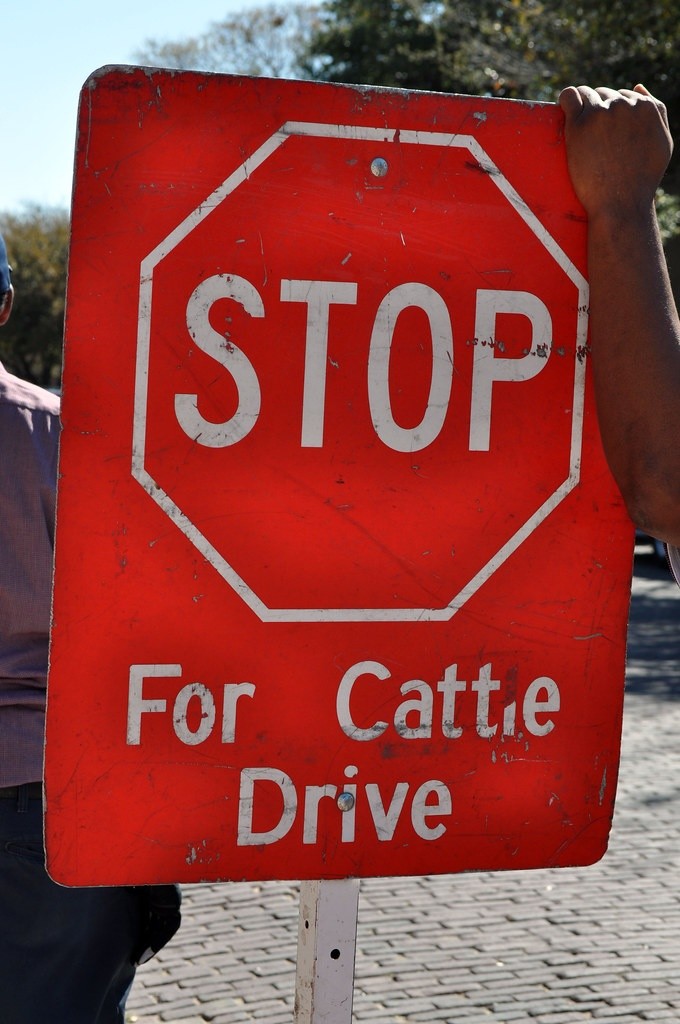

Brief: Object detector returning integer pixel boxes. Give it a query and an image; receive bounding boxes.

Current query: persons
[0,236,180,1024]
[562,82,680,585]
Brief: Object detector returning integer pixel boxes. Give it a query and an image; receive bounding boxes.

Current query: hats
[0,235,13,294]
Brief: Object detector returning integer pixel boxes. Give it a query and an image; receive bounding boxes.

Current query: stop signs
[132,124,593,624]
